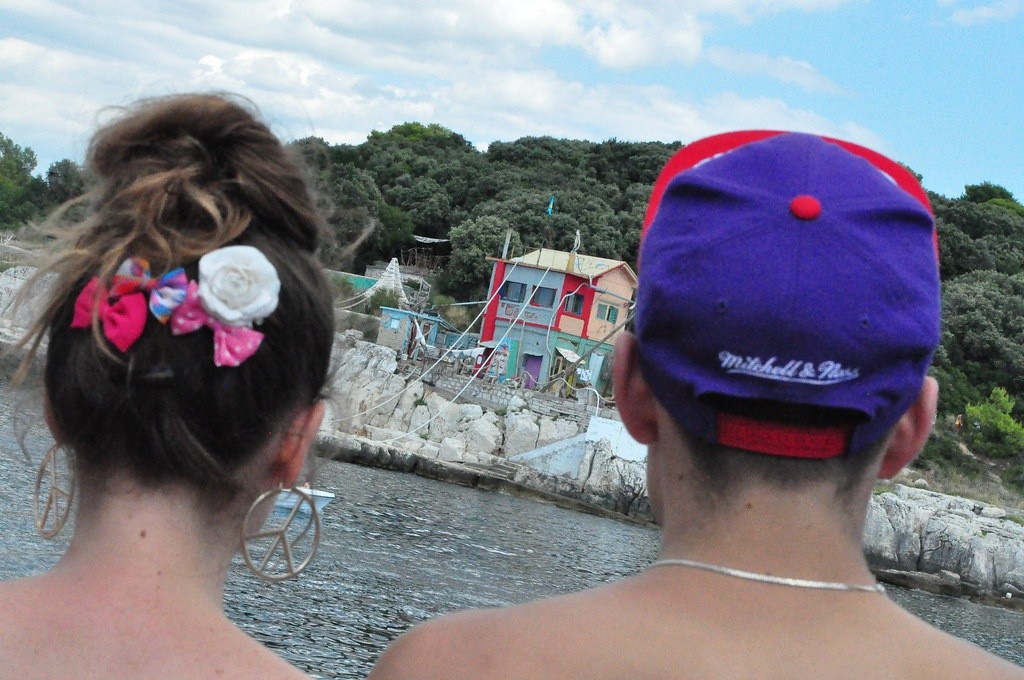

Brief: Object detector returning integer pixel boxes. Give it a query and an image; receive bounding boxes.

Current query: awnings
[556,347,585,364]
[479,340,498,348]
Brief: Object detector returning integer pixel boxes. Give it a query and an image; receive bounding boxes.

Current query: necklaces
[653,560,884,592]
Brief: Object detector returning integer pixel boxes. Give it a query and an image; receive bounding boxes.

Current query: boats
[274,486,336,516]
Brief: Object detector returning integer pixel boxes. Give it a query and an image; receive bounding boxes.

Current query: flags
[548,197,554,215]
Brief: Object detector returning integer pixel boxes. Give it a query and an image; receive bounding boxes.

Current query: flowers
[196,244,283,330]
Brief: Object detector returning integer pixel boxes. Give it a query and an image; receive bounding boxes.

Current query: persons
[370,129,1024,680]
[0,93,334,680]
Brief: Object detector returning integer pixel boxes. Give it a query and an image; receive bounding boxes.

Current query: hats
[636,130,941,457]
